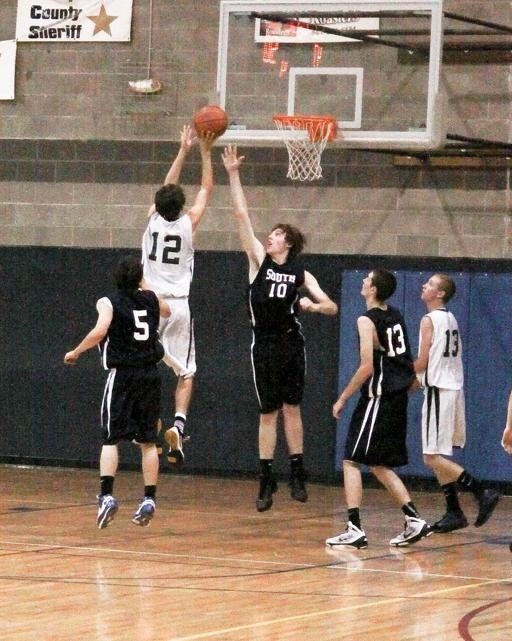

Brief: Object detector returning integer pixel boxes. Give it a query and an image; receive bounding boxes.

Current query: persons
[63,255,172,530]
[131,124,219,465]
[220,141,340,512]
[500,388,512,457]
[324,266,435,550]
[412,271,500,534]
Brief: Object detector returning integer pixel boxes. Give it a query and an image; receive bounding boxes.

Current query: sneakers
[430,511,470,534]
[471,485,500,529]
[325,522,369,550]
[254,477,278,513]
[164,425,187,470]
[154,416,163,459]
[96,493,117,530]
[129,496,156,528]
[289,473,310,502]
[388,513,433,548]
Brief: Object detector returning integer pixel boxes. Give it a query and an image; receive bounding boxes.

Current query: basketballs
[195,106,227,137]
